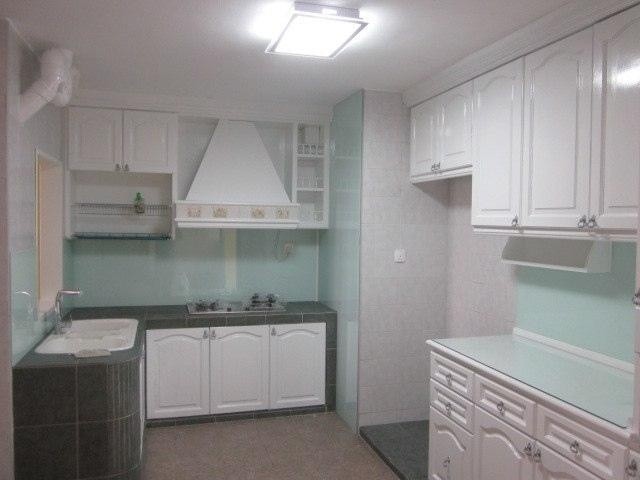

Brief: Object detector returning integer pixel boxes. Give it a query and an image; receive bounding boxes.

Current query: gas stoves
[185,290,286,315]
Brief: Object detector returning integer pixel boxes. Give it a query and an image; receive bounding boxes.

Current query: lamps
[263,3,369,60]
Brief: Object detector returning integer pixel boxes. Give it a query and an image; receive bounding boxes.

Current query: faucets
[14,288,34,326]
[54,290,81,335]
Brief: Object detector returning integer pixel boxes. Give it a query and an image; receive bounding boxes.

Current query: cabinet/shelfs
[271,325,326,410]
[475,372,627,480]
[290,120,330,228]
[147,324,270,419]
[409,80,471,183]
[472,57,519,230]
[65,171,175,244]
[521,3,639,233]
[426,351,474,478]
[67,105,179,174]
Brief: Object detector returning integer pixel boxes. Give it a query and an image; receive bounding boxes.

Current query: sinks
[35,334,134,354]
[74,318,139,333]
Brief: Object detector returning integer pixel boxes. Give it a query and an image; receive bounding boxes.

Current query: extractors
[174,117,300,230]
